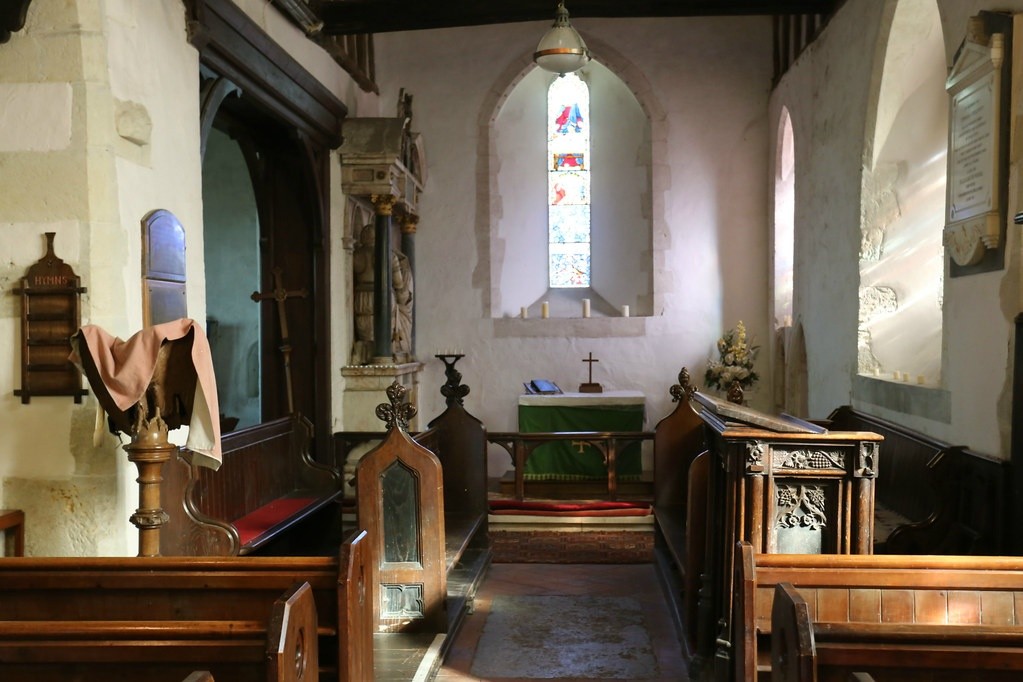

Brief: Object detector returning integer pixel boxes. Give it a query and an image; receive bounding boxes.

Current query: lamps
[534,0,594,77]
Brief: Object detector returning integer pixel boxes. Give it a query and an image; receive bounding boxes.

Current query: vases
[728,380,744,404]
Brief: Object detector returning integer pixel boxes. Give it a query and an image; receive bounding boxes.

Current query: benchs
[159,413,342,558]
[652,368,717,682]
[355,371,488,634]
[0,529,375,682]
[735,541,1023,681]
[816,407,964,555]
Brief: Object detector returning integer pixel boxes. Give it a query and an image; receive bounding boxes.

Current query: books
[531,380,555,395]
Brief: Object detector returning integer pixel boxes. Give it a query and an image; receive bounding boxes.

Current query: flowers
[703,320,762,391]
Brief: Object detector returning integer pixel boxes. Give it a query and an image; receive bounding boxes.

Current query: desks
[518,390,647,481]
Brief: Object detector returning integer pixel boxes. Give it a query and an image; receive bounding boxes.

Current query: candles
[542,301,549,318]
[436,349,464,355]
[521,307,527,318]
[582,299,591,318]
[621,305,629,317]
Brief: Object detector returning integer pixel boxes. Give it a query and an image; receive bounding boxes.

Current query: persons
[351,224,414,366]
